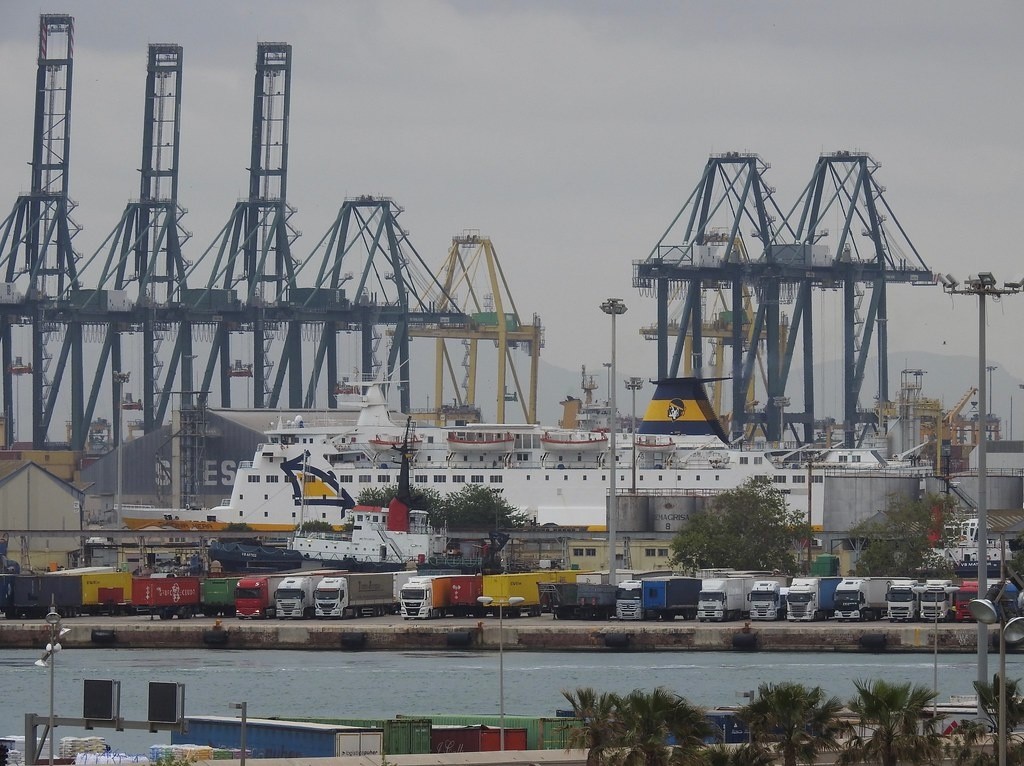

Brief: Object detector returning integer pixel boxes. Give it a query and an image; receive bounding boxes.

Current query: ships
[111,376,935,557]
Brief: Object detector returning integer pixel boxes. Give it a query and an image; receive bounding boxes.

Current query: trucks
[616,579,661,621]
[884,579,1020,623]
[785,576,844,622]
[536,582,616,622]
[745,580,790,622]
[832,576,917,622]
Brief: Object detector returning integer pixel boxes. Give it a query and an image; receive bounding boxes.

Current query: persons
[478,542,489,564]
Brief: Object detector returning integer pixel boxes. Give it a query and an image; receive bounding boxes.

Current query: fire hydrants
[216,619,223,628]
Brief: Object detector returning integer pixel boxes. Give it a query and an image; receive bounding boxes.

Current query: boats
[632,436,678,452]
[538,431,610,454]
[446,430,516,453]
[368,436,423,451]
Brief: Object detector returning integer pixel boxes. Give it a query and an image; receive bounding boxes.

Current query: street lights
[599,297,628,586]
[478,596,524,751]
[772,395,790,447]
[987,364,998,434]
[940,271,1024,724]
[965,580,1021,766]
[800,448,822,565]
[624,376,645,487]
[601,361,614,403]
[35,606,71,766]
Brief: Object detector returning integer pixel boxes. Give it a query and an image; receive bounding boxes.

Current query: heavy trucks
[232,569,348,621]
[313,570,420,620]
[273,575,326,619]
[641,575,707,622]
[697,577,788,626]
[400,568,598,620]
